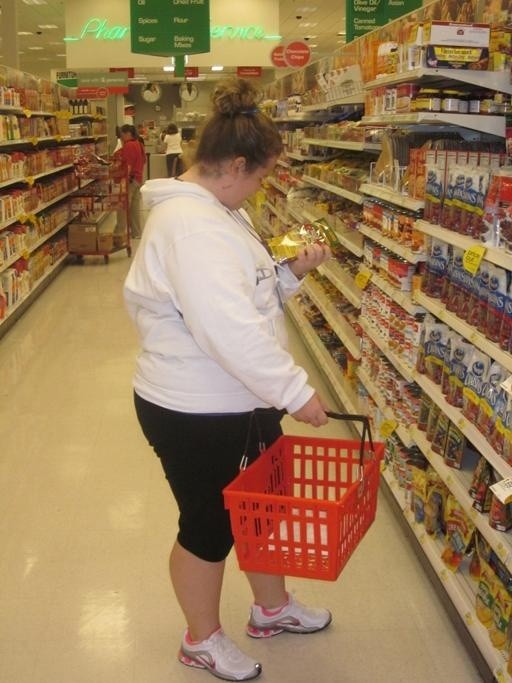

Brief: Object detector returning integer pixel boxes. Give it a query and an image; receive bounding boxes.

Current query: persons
[113,123,184,239]
[121,78,332,681]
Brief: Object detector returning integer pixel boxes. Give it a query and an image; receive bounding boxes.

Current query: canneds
[410,86,511,116]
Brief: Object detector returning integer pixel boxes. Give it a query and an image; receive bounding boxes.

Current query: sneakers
[245,590,332,639]
[176,622,264,682]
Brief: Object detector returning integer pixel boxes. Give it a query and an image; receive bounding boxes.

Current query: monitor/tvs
[182,127,196,142]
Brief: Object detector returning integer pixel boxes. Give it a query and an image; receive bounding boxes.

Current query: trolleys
[65,156,132,263]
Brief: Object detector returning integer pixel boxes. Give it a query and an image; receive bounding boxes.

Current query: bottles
[360,196,426,255]
[68,96,95,137]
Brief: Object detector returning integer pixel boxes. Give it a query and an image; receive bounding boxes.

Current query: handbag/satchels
[218,402,389,583]
